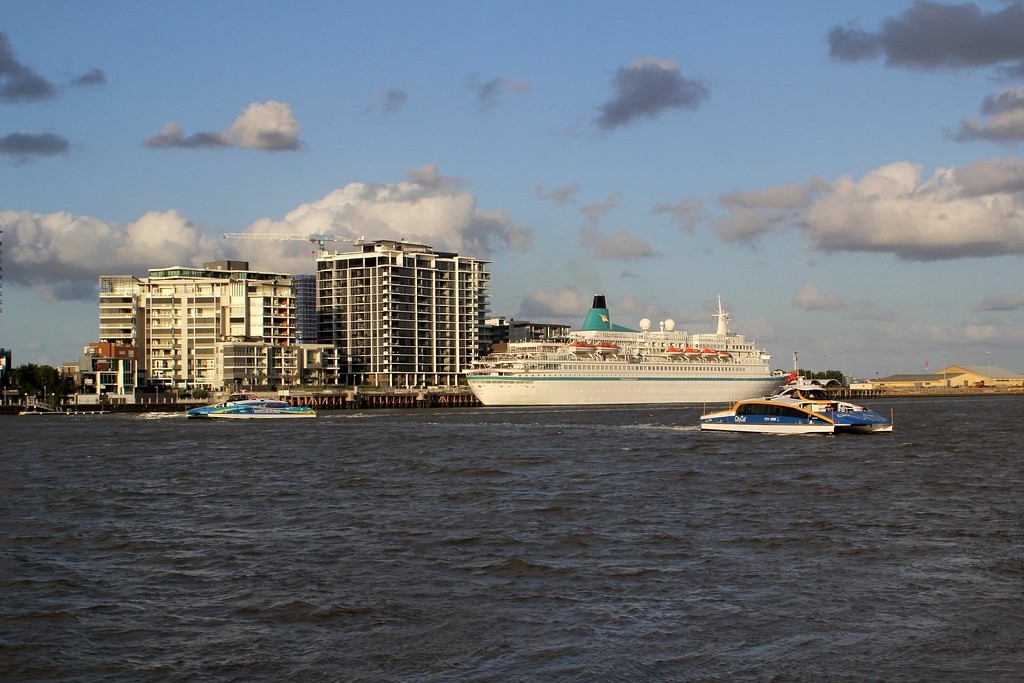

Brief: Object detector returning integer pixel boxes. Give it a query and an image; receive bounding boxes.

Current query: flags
[784,372,796,382]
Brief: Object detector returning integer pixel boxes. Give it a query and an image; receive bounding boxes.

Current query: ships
[459,294,797,408]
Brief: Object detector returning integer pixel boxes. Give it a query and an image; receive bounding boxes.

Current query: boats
[663,347,683,355]
[702,348,718,357]
[185,391,317,419]
[699,370,895,437]
[594,342,621,354]
[568,341,596,355]
[719,351,730,358]
[684,349,701,359]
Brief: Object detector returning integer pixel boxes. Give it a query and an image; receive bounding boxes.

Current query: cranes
[220,229,354,256]
